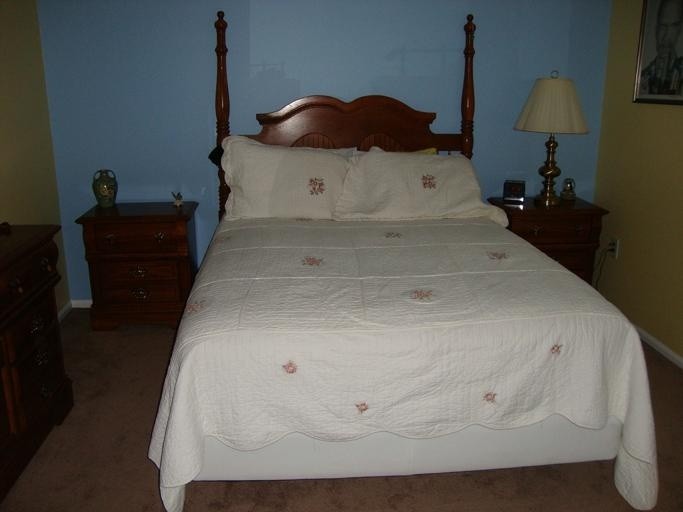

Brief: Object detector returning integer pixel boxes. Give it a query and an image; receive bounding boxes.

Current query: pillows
[220,133,511,226]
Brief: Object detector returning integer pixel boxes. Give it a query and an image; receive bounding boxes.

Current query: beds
[165,9,648,512]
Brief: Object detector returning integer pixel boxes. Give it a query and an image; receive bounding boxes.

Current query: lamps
[510,69,591,208]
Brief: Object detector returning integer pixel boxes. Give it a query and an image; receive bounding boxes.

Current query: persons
[638,0,682,98]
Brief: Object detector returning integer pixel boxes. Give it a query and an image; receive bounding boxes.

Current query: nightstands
[484,193,610,276]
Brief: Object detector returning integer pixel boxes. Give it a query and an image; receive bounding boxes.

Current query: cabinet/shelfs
[1,219,76,508]
[75,198,198,328]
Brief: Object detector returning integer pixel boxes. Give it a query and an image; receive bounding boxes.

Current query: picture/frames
[629,0,682,107]
